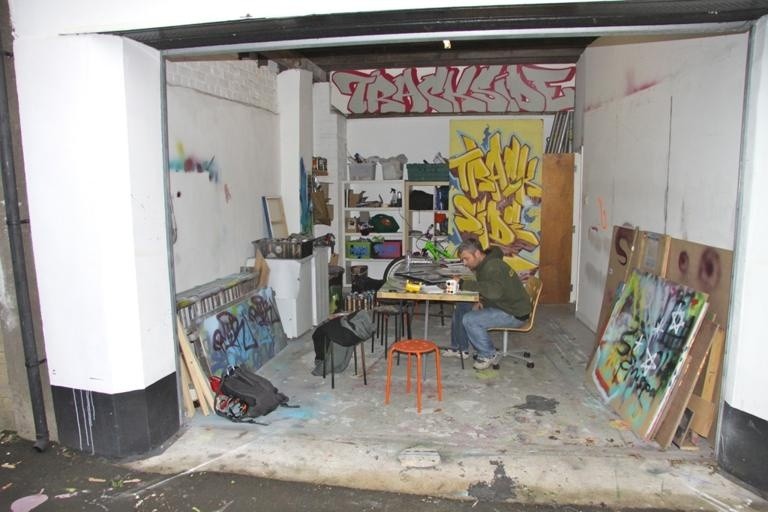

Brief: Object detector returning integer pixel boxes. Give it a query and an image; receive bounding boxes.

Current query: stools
[383,336,442,412]
[370,302,415,354]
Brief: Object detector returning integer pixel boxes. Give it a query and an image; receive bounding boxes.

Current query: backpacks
[213,365,300,427]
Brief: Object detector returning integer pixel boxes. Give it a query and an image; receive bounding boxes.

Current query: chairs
[482,273,545,372]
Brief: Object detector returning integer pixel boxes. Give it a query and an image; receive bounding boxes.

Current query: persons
[442,239,532,370]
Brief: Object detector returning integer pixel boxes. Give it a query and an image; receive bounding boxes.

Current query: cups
[445,279,460,295]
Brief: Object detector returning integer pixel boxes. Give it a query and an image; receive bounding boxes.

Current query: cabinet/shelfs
[342,179,450,290]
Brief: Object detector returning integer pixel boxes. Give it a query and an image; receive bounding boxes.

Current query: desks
[375,268,479,368]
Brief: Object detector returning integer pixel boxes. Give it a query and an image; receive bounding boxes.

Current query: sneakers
[439,347,471,360]
[471,349,500,370]
